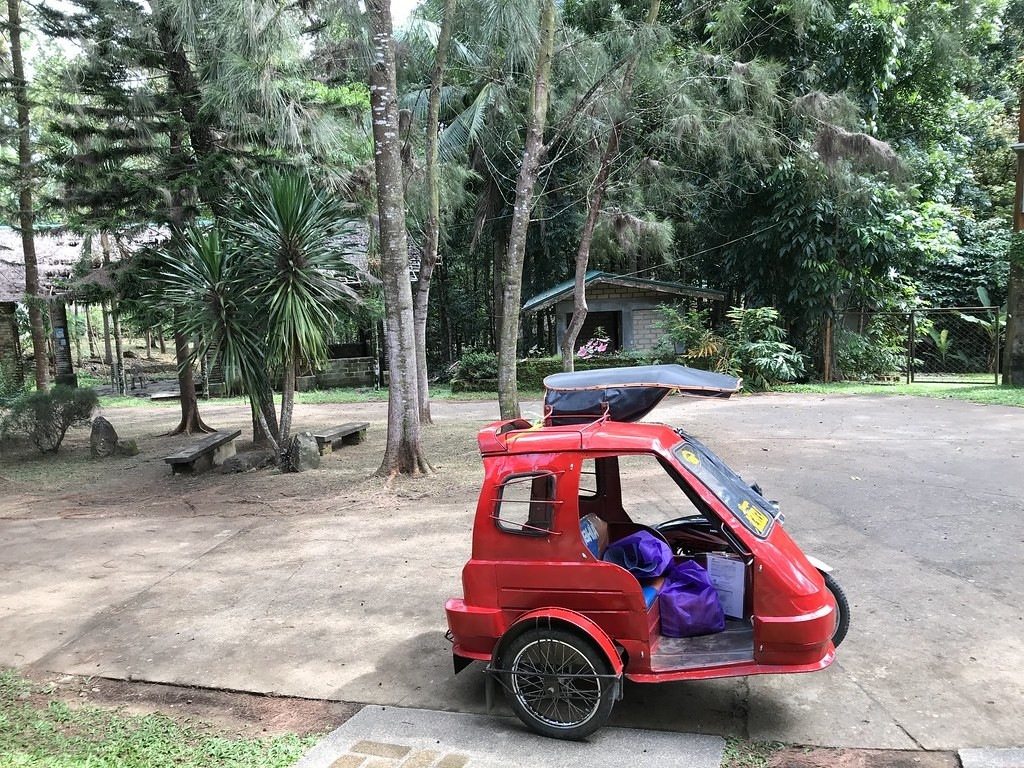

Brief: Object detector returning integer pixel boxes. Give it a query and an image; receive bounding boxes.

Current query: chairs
[579,513,665,612]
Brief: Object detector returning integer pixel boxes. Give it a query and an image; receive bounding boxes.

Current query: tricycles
[445,363,852,743]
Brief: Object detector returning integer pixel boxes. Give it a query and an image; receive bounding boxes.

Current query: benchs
[165,430,241,475]
[313,422,370,456]
